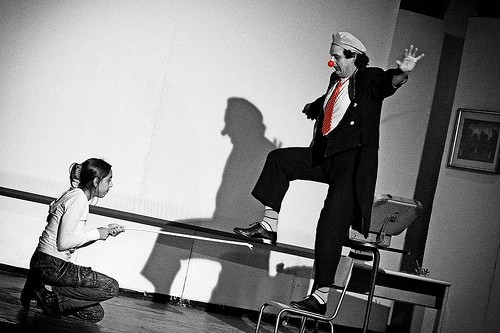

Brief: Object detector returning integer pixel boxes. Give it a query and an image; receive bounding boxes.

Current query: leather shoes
[233,223,277,247]
[291,295,328,316]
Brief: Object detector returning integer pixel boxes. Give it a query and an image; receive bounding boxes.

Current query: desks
[348,263,451,333]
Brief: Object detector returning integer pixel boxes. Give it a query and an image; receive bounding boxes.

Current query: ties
[321,77,348,135]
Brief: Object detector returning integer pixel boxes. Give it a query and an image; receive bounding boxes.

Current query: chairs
[255,256,354,333]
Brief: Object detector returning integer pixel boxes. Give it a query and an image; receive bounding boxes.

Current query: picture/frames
[446,108,500,174]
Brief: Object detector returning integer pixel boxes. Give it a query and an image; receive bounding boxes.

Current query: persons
[20,158,124,323]
[447,108,500,175]
[232,32,424,315]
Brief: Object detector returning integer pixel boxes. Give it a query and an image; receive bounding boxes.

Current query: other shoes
[20,273,40,310]
[32,283,53,314]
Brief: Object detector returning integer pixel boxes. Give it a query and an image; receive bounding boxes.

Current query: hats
[332,32,368,54]
